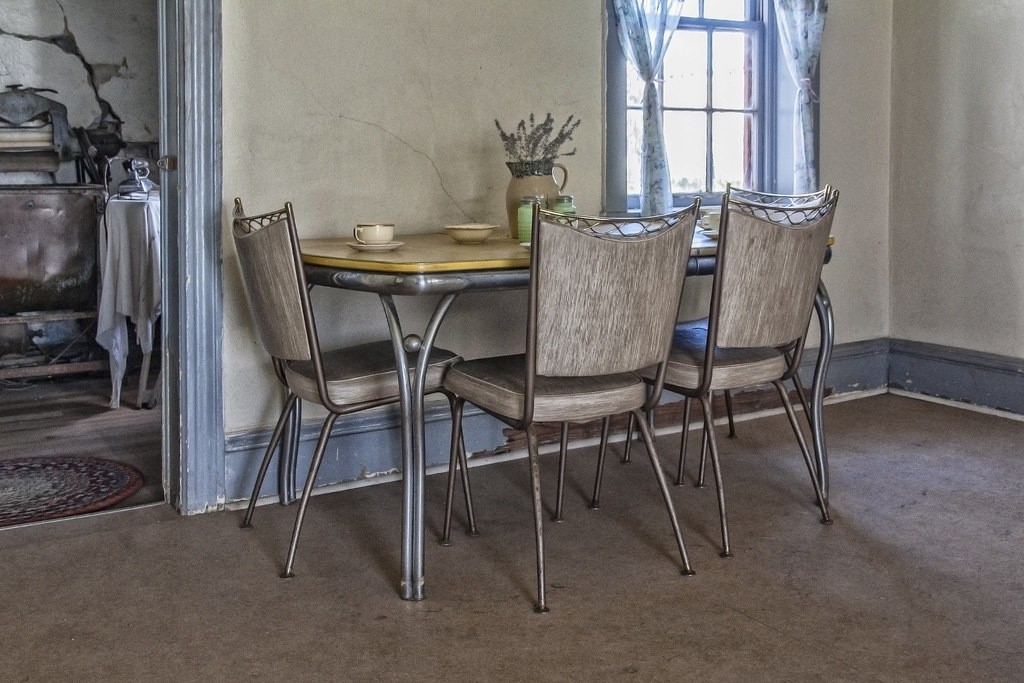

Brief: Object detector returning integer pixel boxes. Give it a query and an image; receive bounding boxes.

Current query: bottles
[551,196,577,214]
[518,196,542,242]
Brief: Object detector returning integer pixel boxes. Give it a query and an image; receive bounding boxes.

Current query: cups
[354,223,395,244]
[701,212,722,230]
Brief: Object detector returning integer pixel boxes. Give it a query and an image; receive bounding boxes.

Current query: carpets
[0,455,145,526]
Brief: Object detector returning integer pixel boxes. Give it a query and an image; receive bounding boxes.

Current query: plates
[700,230,719,239]
[519,242,531,249]
[345,240,405,253]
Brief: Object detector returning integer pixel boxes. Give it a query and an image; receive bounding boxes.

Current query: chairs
[441,195,702,614]
[619,183,834,489]
[591,178,843,559]
[230,195,481,579]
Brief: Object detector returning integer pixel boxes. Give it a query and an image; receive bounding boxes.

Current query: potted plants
[493,112,584,239]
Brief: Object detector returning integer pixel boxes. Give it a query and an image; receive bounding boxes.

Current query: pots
[1,84,59,148]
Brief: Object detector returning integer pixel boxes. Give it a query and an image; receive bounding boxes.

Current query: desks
[94,194,161,408]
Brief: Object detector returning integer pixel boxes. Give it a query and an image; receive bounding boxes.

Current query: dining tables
[279,223,835,601]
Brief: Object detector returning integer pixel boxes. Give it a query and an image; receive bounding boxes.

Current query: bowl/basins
[444,224,498,245]
